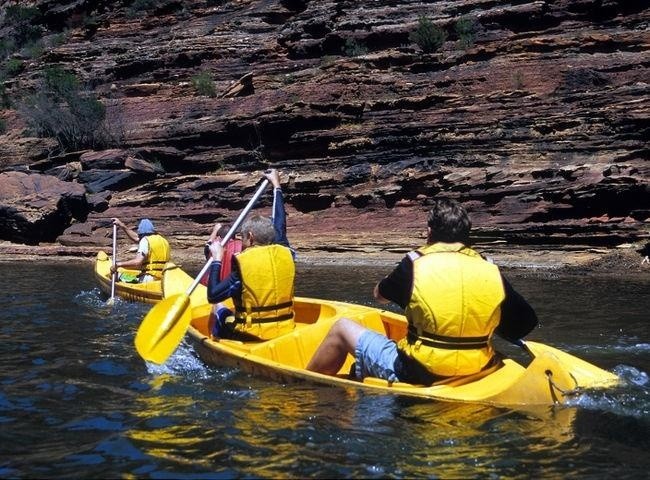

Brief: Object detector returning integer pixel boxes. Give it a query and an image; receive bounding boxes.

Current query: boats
[161,263,625,411]
[94,250,162,305]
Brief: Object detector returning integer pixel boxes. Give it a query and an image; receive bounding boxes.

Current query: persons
[199,224,244,288]
[207,168,298,340]
[109,216,170,284]
[305,201,538,385]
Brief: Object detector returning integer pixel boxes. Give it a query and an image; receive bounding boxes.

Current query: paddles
[134,170,270,366]
[490,333,535,368]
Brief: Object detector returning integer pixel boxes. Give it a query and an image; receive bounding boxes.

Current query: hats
[137,218,156,234]
